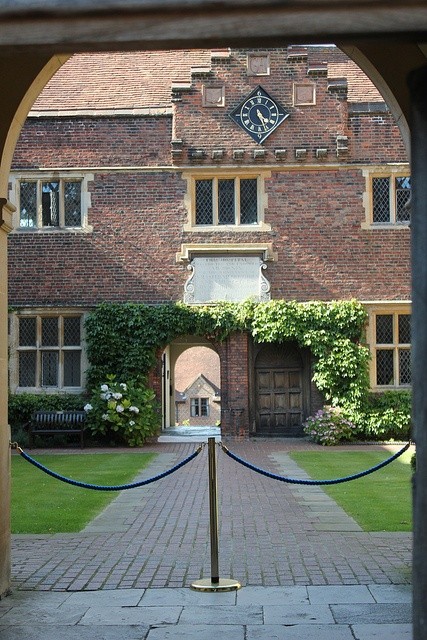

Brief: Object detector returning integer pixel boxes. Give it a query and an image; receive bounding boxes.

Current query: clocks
[228,84,291,146]
